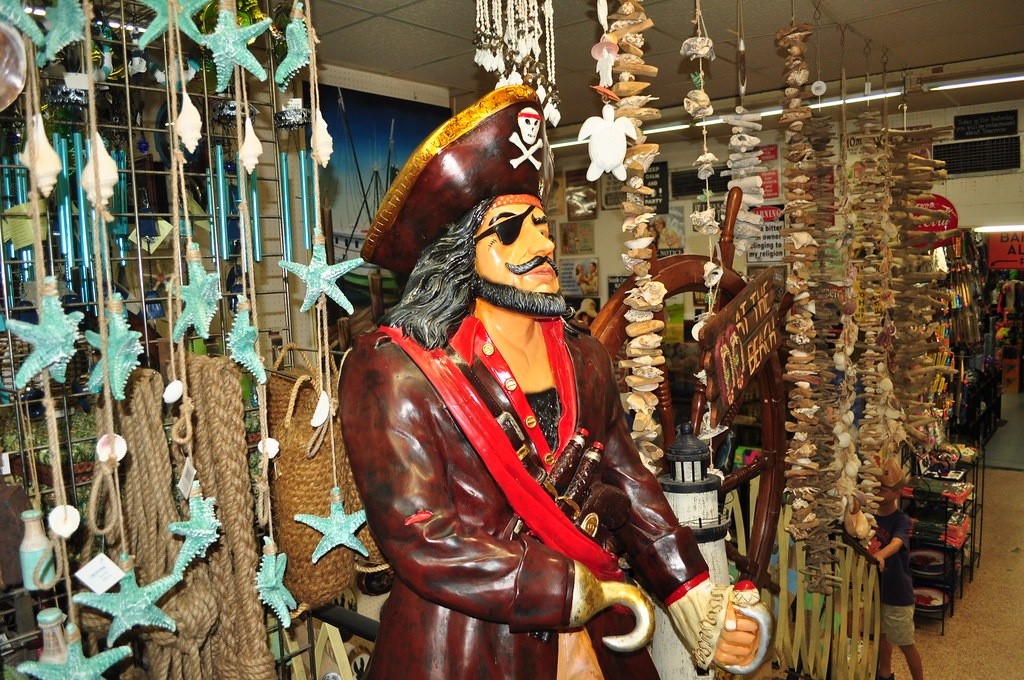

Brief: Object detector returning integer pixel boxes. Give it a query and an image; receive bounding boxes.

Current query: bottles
[973,306,981,342]
[942,325,949,349]
[941,319,947,338]
[948,402,954,420]
[950,319,958,347]
[963,309,973,341]
[966,265,984,305]
[961,266,971,307]
[973,266,981,290]
[942,353,954,380]
[956,268,963,308]
[950,268,956,308]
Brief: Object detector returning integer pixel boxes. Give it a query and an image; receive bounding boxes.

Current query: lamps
[922,69,1024,93]
[548,86,906,149]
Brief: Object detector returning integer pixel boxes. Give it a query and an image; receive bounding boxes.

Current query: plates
[909,549,945,575]
[913,588,949,612]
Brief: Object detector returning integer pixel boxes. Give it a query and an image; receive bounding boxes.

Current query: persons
[870,485,927,680]
[337,81,776,680]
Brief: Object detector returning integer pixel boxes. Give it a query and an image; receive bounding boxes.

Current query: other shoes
[879,672,894,680]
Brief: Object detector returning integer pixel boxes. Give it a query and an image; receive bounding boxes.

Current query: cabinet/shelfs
[899,445,986,634]
[947,363,1003,448]
